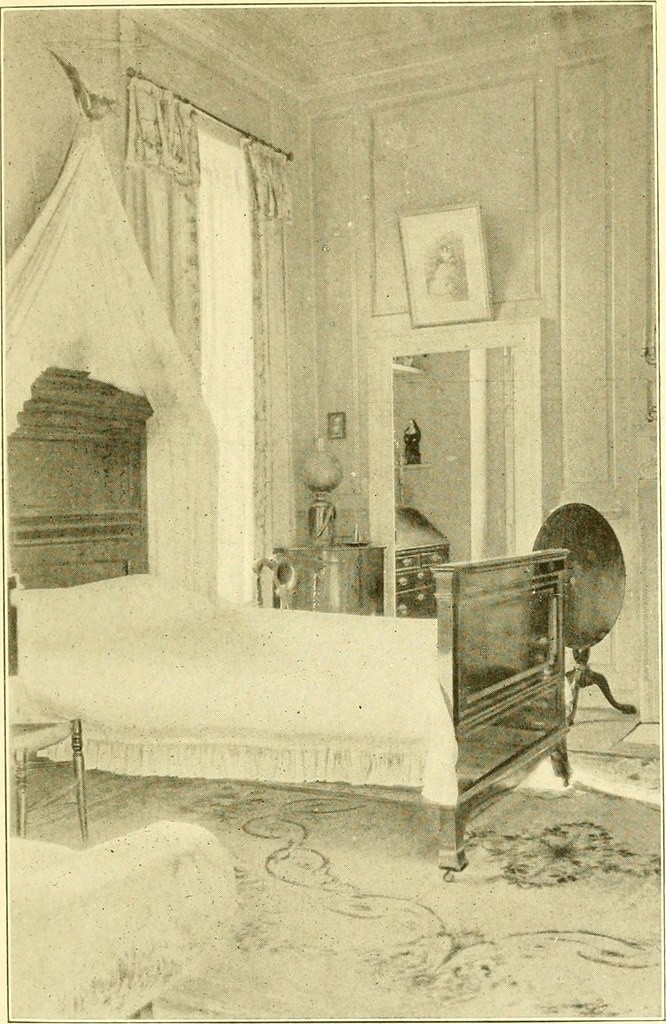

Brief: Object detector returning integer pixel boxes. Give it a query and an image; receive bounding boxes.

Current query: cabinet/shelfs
[269,544,385,619]
[394,507,449,619]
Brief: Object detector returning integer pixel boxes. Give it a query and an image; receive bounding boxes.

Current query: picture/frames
[327,411,347,440]
[396,204,495,328]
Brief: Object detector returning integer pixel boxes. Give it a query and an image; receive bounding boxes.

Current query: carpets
[8,754,664,1020]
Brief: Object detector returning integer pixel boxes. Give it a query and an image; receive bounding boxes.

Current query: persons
[403,419,421,458]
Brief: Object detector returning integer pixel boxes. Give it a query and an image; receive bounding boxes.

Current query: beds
[4,364,575,867]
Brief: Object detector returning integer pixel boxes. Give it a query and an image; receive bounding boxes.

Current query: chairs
[4,572,90,844]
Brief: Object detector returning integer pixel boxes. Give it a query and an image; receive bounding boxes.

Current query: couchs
[8,817,240,1022]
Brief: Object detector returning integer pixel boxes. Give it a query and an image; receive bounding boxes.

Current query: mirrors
[363,316,542,621]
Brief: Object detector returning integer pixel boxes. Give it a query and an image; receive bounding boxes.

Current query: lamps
[298,451,342,548]
